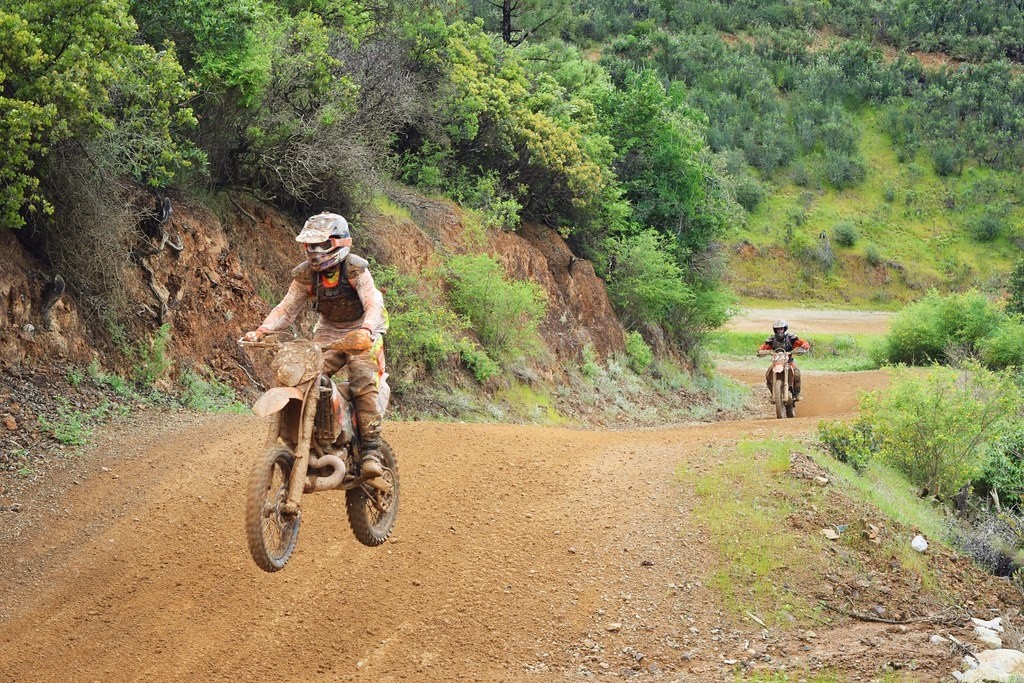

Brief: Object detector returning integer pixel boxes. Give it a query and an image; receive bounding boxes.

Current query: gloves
[243,330,263,351]
[344,329,371,355]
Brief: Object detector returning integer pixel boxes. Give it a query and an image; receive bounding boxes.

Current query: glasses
[307,238,353,253]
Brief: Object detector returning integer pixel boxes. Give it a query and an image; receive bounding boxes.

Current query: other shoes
[794,393,803,401]
[361,449,382,477]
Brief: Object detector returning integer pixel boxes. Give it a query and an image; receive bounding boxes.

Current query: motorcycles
[756,349,805,419]
[237,330,402,570]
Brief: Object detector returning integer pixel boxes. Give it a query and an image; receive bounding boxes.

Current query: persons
[757,320,810,401]
[243,211,389,479]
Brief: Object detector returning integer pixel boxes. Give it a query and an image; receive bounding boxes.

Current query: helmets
[773,319,788,339]
[296,213,352,271]
